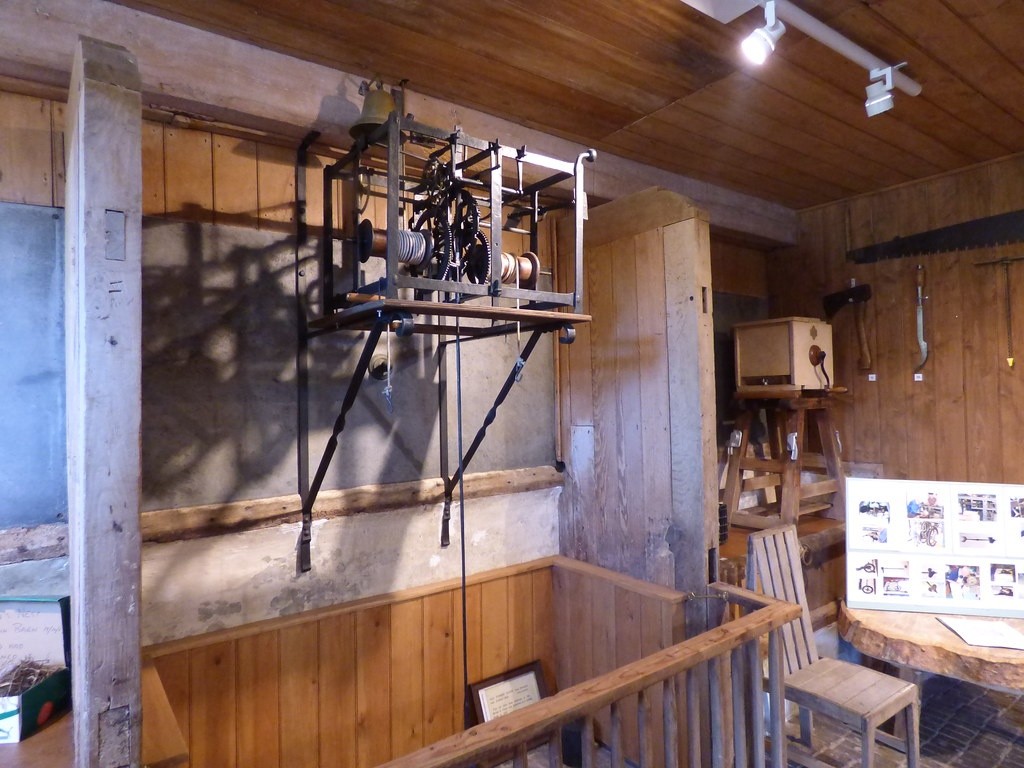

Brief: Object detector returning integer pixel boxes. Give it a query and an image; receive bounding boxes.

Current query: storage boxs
[0,592,71,746]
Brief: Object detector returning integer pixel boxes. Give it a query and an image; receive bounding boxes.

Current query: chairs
[744,526,921,768]
